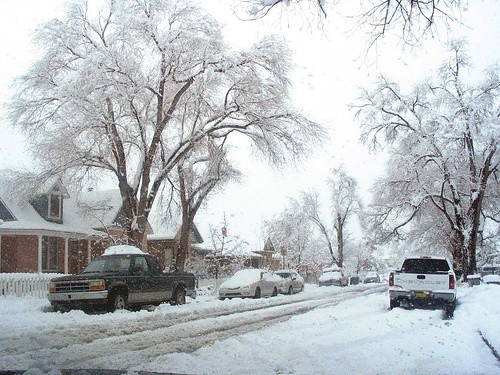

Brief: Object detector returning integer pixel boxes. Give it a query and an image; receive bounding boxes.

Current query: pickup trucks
[47,253,199,313]
[388,255,457,313]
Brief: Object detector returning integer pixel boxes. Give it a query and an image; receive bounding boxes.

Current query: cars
[363,273,381,283]
[319,267,350,288]
[274,269,305,296]
[218,268,281,298]
